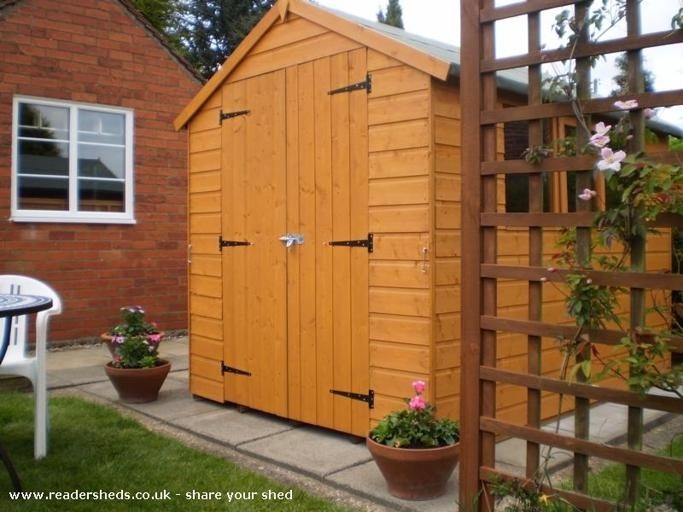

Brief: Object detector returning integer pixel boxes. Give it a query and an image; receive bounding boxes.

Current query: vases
[102,331,161,357]
[366,432,459,500]
[105,360,171,404]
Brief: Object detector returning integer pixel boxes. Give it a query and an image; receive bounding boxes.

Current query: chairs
[0,275,62,461]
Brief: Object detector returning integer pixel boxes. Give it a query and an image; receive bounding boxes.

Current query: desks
[0,294,53,497]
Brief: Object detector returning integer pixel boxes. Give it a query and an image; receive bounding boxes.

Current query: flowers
[370,381,459,448]
[107,306,159,337]
[112,334,161,369]
